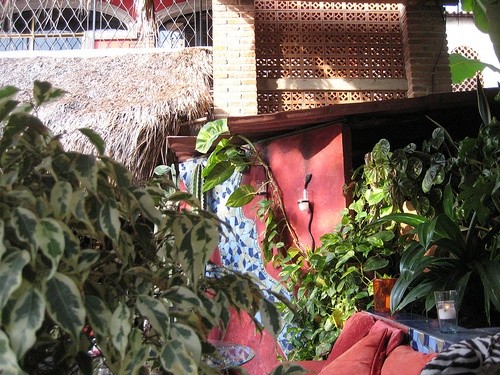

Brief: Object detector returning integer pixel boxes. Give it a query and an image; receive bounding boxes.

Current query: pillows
[311,312,438,374]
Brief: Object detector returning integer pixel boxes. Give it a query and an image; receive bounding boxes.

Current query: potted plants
[372,271,399,317]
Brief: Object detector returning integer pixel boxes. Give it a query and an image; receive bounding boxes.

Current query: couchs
[208,299,326,375]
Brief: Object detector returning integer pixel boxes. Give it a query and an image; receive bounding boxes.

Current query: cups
[372,278,396,314]
[434,291,459,334]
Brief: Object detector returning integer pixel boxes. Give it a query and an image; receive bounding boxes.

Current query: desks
[360,309,497,354]
[201,339,255,375]
